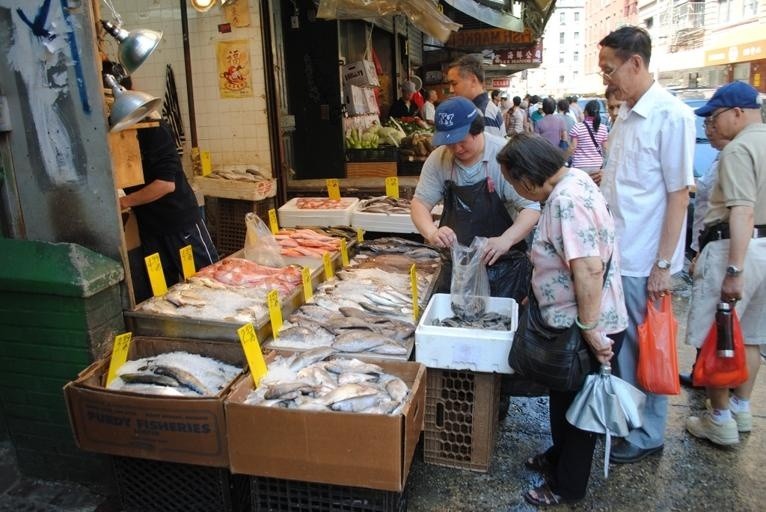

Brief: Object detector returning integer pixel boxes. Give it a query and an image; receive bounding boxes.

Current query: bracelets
[573,315,603,331]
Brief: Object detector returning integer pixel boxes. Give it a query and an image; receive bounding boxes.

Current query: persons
[692,79,766,448]
[490,89,500,106]
[500,94,506,111]
[448,55,508,138]
[496,133,630,506]
[599,25,698,465]
[572,97,583,118]
[681,117,729,384]
[534,97,567,147]
[531,104,545,129]
[561,100,607,173]
[506,96,527,136]
[528,95,542,115]
[423,89,438,125]
[388,82,428,128]
[409,97,541,298]
[604,89,624,124]
[519,93,529,108]
[566,96,583,120]
[96,60,221,289]
[554,99,576,134]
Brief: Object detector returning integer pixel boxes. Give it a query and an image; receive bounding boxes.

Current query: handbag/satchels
[508,202,615,393]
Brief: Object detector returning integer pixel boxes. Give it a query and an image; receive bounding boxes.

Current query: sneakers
[686,415,740,446]
[706,397,753,433]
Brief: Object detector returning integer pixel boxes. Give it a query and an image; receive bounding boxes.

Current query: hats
[694,80,761,117]
[402,81,416,92]
[431,95,479,146]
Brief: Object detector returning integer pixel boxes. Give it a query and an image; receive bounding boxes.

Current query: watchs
[656,259,672,269]
[725,266,745,275]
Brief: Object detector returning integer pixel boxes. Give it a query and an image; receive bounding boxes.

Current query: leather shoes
[678,373,703,390]
[610,438,664,464]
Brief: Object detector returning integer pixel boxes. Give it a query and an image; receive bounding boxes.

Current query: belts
[707,225,765,240]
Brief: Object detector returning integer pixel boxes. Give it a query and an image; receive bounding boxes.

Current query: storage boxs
[214,197,268,259]
[223,349,427,493]
[345,142,396,162]
[249,475,407,511]
[277,196,360,227]
[107,454,251,512]
[61,335,264,470]
[344,84,365,116]
[341,60,381,88]
[423,366,503,472]
[362,87,381,115]
[413,292,521,376]
[196,174,278,202]
[351,199,443,233]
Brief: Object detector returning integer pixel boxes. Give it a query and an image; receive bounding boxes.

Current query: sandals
[525,446,554,469]
[526,479,586,505]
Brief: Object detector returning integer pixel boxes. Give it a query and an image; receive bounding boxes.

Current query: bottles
[715,301,735,357]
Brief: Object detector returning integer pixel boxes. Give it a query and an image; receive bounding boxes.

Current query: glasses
[598,57,629,81]
[707,106,744,125]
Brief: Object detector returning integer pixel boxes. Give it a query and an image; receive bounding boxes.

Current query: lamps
[189,0,217,12]
[98,17,163,77]
[103,72,161,134]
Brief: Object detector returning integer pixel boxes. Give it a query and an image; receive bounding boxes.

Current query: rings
[660,293,665,297]
[729,298,737,302]
